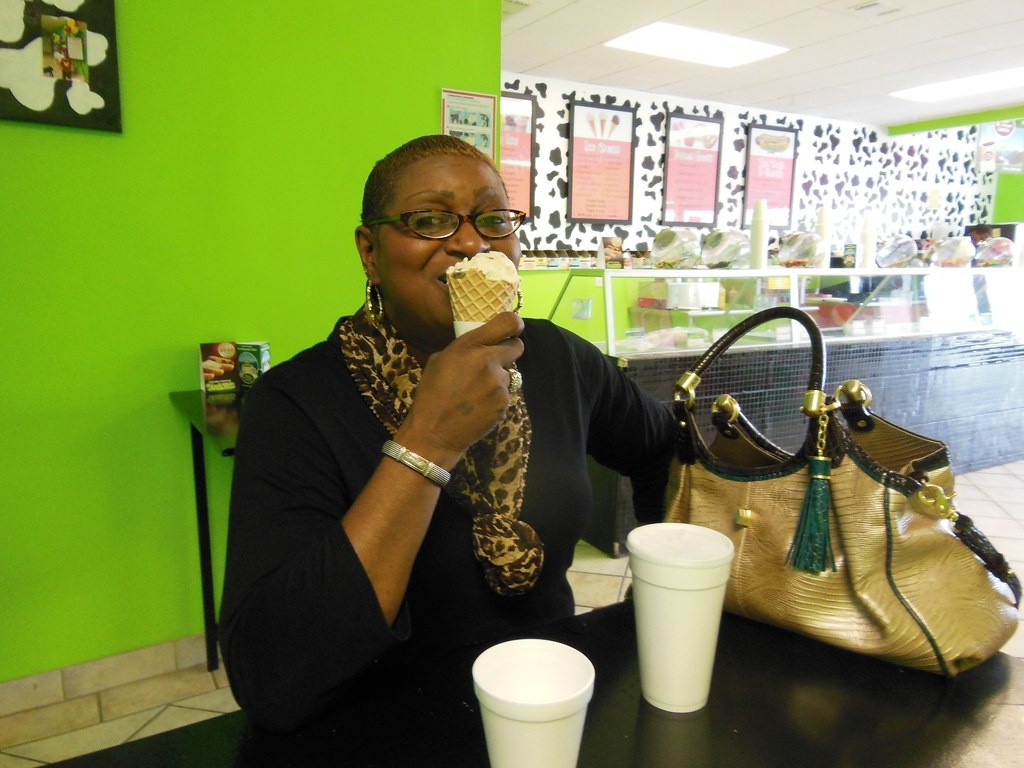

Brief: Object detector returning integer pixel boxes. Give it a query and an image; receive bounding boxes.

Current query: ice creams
[582,111,620,138]
[447,251,518,323]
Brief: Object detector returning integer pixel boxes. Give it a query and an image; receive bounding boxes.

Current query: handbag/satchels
[663,307,1022,675]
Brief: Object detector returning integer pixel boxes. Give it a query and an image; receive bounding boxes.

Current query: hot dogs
[756,133,790,152]
[201,356,234,379]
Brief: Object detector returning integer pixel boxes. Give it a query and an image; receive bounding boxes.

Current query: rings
[508,369,523,392]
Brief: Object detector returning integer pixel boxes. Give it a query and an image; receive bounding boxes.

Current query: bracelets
[383,440,451,488]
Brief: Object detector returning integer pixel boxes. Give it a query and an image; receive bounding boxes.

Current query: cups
[750,199,769,269]
[812,205,830,269]
[472,638,596,767]
[626,523,735,714]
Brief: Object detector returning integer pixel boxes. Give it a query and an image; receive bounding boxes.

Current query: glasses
[363,208,526,239]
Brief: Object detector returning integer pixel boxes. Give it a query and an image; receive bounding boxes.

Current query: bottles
[778,231,825,269]
[701,228,748,270]
[650,229,701,269]
[875,234,1016,269]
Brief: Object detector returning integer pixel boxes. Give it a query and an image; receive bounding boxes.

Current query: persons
[967,224,995,289]
[217,134,681,731]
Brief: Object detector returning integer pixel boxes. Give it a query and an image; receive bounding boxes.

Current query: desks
[32,593,1024,768]
[168,388,245,675]
